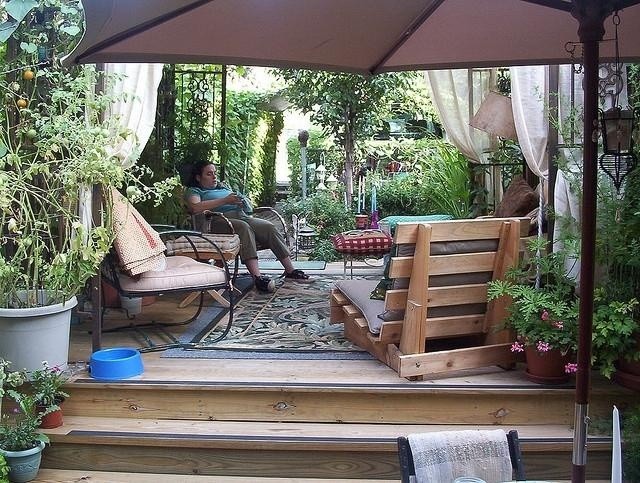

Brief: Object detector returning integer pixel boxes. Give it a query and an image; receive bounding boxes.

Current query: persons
[184,160,316,293]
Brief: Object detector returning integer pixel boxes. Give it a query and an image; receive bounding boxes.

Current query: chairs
[328,216,532,383]
[472,174,544,237]
[397,428,525,483]
[88,183,233,353]
[181,206,291,288]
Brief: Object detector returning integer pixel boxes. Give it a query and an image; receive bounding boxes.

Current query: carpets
[227,260,326,271]
[159,273,387,360]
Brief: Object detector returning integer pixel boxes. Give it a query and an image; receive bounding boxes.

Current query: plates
[300,230,314,233]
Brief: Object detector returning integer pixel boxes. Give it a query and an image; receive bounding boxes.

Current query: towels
[407,428,513,483]
[107,185,168,277]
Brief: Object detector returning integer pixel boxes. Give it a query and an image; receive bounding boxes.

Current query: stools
[332,229,392,279]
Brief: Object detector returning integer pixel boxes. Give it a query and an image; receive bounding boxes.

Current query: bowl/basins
[89,348,144,380]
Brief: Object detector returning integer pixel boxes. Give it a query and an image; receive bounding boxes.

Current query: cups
[356,215,368,230]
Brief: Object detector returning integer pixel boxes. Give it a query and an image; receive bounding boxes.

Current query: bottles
[379,219,390,235]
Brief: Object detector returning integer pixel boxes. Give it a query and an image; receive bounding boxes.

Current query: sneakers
[282,269,316,284]
[252,275,275,292]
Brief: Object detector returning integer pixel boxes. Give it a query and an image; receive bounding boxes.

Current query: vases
[35,396,65,429]
[519,335,565,378]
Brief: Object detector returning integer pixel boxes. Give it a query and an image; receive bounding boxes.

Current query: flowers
[486,239,578,381]
[22,359,68,407]
[0,355,31,417]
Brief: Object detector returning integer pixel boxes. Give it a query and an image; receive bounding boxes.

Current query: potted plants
[0,65,140,382]
[590,285,639,380]
[0,404,62,483]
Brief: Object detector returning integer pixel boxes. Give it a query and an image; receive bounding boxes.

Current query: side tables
[377,213,455,276]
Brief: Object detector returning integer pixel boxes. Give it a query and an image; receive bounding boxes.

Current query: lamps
[467,89,518,141]
[295,212,315,261]
[591,14,639,195]
